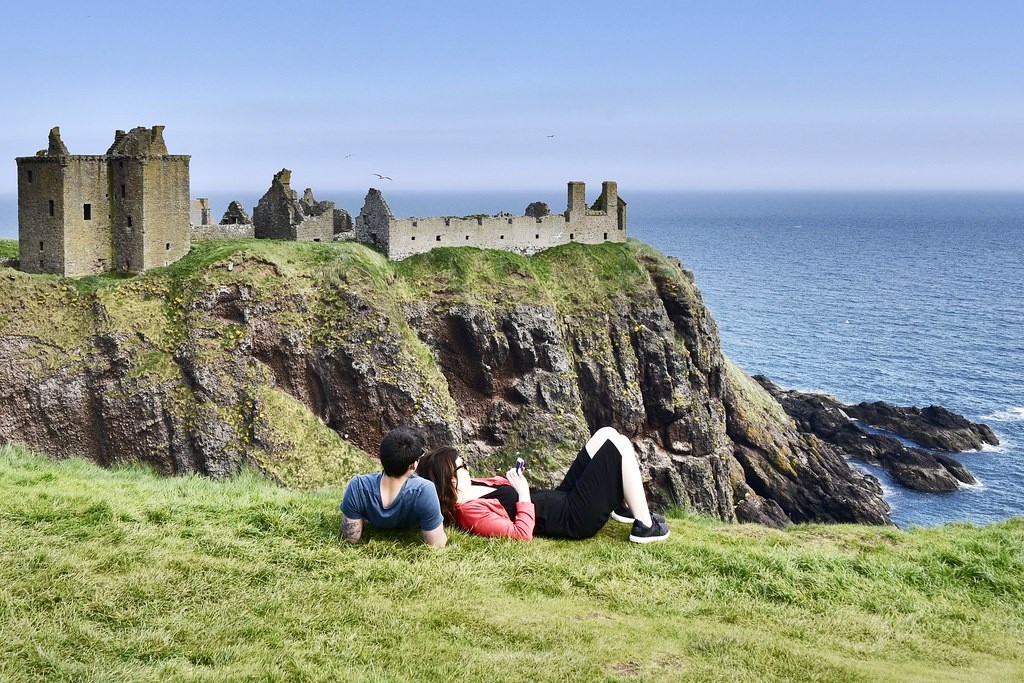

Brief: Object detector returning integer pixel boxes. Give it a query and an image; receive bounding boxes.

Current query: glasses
[417,448,426,461]
[453,459,467,473]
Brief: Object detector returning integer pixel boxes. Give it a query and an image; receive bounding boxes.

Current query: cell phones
[515,458,524,475]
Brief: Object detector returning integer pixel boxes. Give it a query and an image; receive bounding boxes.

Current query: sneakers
[610,502,667,525]
[629,519,671,544]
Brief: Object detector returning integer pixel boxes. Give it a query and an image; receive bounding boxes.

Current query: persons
[340,427,447,548]
[417,427,671,543]
